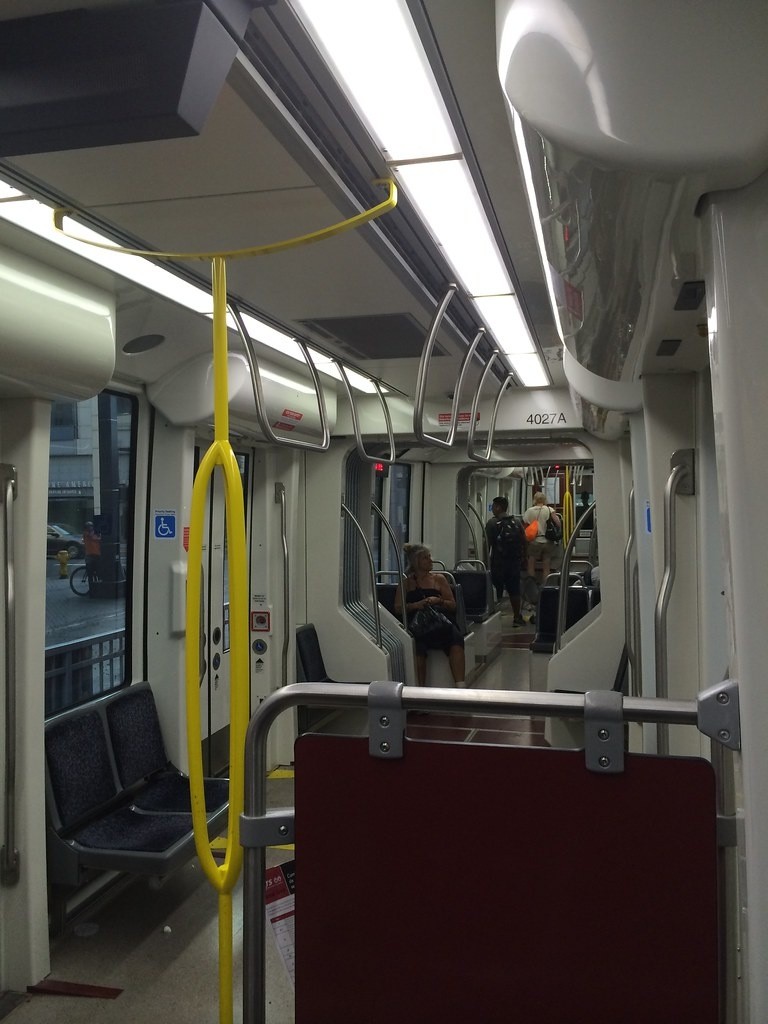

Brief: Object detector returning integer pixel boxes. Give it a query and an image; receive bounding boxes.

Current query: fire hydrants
[58,550,69,579]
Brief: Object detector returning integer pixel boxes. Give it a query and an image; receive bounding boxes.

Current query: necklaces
[418,573,429,581]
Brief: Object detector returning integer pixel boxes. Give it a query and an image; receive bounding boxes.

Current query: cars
[47,522,85,560]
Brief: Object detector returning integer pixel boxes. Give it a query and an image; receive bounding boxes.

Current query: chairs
[46,554,601,923]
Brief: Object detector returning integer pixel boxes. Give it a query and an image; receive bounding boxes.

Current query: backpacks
[495,514,524,563]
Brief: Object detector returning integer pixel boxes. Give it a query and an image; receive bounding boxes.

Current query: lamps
[2,183,388,395]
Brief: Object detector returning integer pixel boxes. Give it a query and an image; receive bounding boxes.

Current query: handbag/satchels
[409,599,454,643]
[524,520,538,542]
[545,506,564,541]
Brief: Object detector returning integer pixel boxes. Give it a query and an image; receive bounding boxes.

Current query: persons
[394,542,467,689]
[483,496,531,626]
[576,491,594,529]
[82,521,101,594]
[522,490,562,585]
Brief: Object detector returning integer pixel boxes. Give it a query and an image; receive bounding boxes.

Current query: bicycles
[70,562,98,597]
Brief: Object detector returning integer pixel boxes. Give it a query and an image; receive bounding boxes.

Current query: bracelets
[439,595,444,607]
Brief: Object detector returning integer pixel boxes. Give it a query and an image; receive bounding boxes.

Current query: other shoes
[512,614,527,627]
